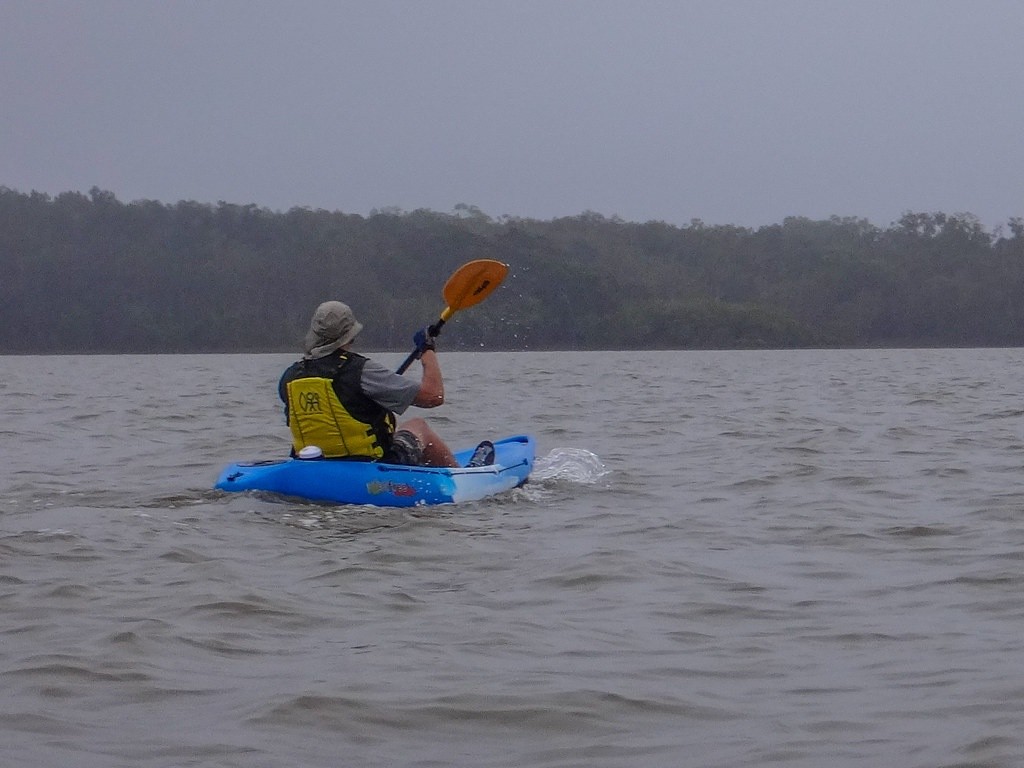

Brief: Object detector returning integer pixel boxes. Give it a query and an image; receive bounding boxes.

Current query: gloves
[413,325,436,360]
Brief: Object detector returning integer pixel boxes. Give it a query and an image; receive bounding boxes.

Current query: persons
[278,300,496,471]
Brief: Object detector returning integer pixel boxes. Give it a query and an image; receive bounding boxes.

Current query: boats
[212,434,537,509]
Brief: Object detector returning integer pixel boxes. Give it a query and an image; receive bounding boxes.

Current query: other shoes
[464,440,495,468]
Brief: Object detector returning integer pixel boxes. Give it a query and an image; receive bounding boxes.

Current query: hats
[303,301,363,359]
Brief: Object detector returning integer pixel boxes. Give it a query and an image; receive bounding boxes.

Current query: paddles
[394,258,509,375]
